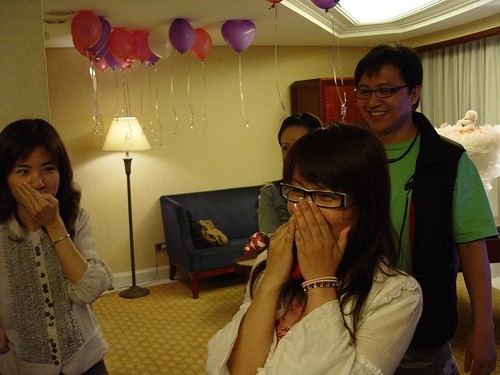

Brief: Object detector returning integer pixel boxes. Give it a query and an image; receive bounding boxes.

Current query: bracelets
[53,233,71,247]
[302,276,339,291]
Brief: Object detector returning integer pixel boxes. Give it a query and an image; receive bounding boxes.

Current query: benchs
[160,185,263,299]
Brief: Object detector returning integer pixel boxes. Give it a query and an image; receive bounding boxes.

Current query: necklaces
[388,130,418,164]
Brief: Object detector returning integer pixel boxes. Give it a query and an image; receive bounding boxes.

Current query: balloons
[73,37,132,71]
[87,16,110,53]
[266,0,283,3]
[190,28,212,59]
[311,0,340,9]
[71,10,101,47]
[169,18,195,54]
[221,20,257,53]
[108,27,133,66]
[147,24,175,58]
[144,52,159,64]
[132,30,148,62]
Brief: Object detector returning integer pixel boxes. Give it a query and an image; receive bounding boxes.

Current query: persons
[0,117,114,375]
[203,122,423,375]
[256,112,324,235]
[353,42,500,375]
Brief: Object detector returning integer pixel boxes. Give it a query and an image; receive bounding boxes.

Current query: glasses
[280,183,359,208]
[354,86,406,99]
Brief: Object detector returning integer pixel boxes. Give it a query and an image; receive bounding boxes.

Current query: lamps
[101,117,151,299]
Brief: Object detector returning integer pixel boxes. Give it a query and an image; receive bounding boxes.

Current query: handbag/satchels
[186,210,228,249]
